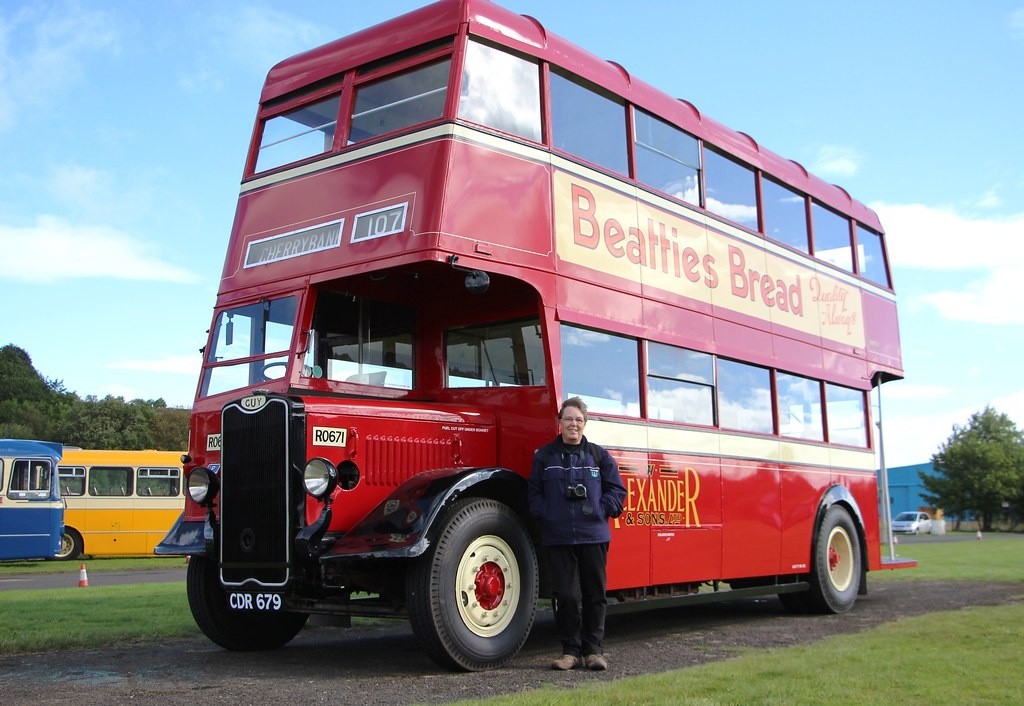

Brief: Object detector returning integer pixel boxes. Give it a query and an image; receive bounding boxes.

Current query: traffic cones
[79,563,89,587]
[893,533,898,544]
[186,556,190,563]
[977,529,982,539]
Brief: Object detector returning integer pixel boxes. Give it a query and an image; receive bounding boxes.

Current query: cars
[891,512,932,535]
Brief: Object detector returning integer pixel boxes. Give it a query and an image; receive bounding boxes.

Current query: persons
[528,394,626,671]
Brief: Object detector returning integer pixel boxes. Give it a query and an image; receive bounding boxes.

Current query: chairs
[64,485,179,497]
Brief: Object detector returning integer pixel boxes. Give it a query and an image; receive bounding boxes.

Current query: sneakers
[552,654,584,670]
[585,654,607,670]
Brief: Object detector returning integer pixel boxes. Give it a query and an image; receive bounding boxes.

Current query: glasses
[560,416,584,424]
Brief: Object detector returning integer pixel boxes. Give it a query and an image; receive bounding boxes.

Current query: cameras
[566,484,588,501]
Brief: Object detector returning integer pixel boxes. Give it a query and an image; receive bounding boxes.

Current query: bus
[35,446,189,561]
[155,1,919,672]
[0,439,65,559]
